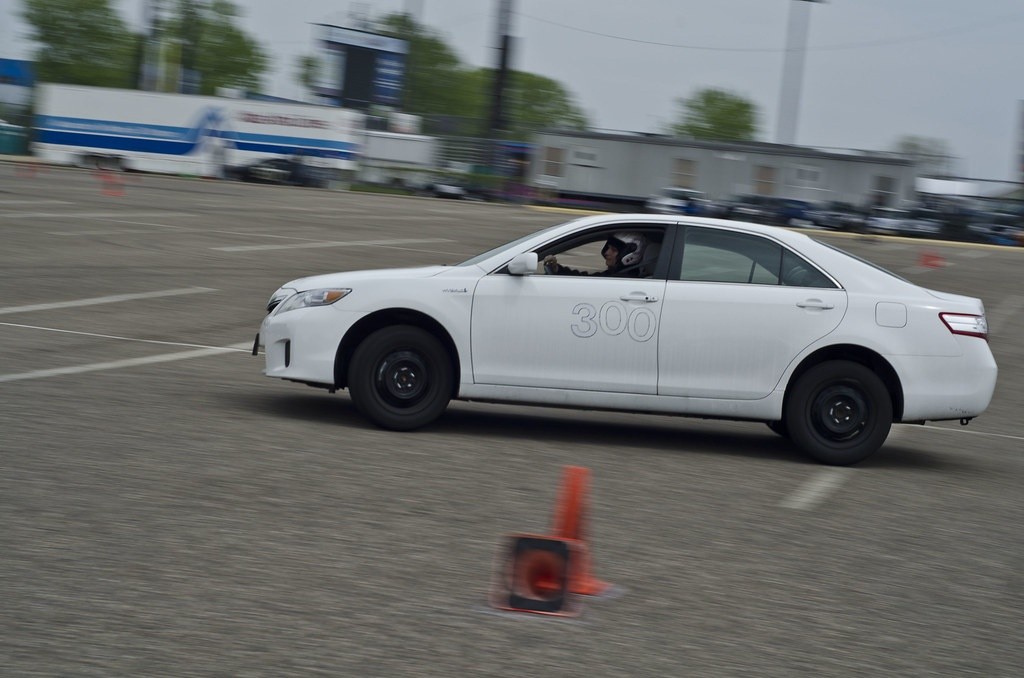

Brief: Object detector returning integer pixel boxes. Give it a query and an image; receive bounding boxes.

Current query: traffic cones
[548,466,610,596]
[487,529,578,618]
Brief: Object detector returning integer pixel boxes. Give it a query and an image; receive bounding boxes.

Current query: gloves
[543,255,558,274]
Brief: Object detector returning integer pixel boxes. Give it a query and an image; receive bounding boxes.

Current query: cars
[247,159,304,187]
[252,214,999,464]
[647,186,1024,245]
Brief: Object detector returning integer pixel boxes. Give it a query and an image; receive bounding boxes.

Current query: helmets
[601,233,646,267]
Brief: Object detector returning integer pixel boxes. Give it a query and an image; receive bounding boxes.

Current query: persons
[544,231,649,277]
